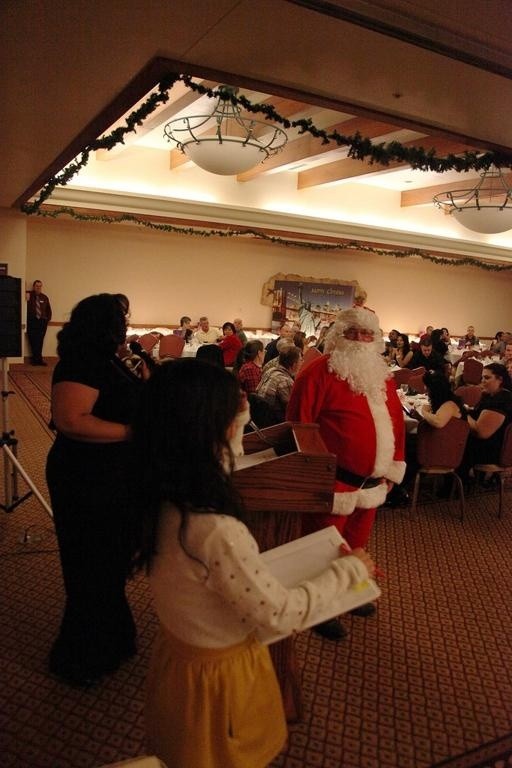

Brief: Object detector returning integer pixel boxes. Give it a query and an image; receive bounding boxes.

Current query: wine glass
[390,360,396,370]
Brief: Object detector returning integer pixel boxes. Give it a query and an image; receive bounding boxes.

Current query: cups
[203,337,208,343]
[400,384,409,394]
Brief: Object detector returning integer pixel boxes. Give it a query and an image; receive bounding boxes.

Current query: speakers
[0,274,22,357]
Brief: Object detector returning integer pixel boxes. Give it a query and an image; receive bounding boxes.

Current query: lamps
[162,85,288,176]
[433,152,512,233]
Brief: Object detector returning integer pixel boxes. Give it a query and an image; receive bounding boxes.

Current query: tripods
[0,357,54,521]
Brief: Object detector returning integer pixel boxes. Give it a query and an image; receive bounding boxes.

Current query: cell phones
[403,405,411,415]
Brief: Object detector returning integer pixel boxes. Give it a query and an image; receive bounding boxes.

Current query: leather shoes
[385,494,411,508]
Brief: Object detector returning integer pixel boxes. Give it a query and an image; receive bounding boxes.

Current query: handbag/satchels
[247,392,287,427]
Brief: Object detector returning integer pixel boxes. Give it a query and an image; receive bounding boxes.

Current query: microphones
[129,342,155,370]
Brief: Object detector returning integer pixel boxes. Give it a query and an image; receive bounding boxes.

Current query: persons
[117,356,378,768]
[297,284,316,337]
[179,317,308,409]
[381,326,512,488]
[287,305,407,640]
[25,279,52,367]
[44,291,151,689]
